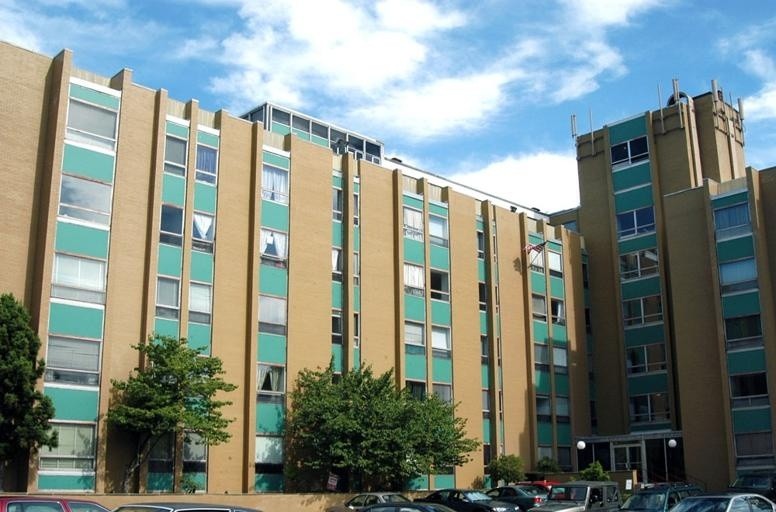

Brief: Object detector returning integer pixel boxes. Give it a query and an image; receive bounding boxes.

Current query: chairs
[352,497,387,507]
[664,496,678,509]
[573,487,601,503]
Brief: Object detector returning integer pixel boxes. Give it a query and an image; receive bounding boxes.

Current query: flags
[522,240,546,254]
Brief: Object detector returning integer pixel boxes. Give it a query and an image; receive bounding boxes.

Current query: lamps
[577,439,586,450]
[668,435,677,448]
